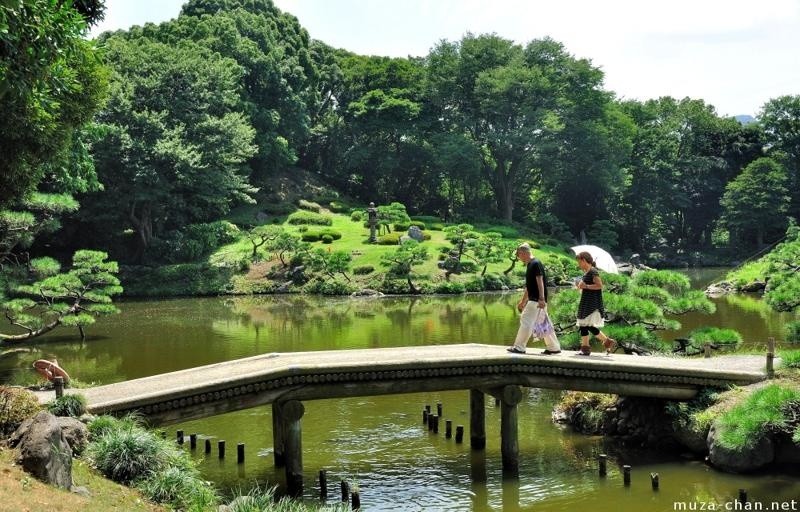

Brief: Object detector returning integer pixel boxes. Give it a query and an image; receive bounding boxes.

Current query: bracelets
[538,296,545,301]
[585,284,590,290]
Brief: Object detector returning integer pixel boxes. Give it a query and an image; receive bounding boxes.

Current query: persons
[574,251,616,355]
[505,242,562,355]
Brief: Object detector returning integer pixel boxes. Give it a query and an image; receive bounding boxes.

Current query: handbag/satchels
[533,309,553,338]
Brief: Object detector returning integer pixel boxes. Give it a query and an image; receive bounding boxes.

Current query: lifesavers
[32,359,71,383]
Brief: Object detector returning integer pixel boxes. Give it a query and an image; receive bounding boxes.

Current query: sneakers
[507,347,525,354]
[541,349,562,355]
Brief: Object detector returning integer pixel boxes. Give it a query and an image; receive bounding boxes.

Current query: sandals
[576,346,590,355]
[604,338,614,355]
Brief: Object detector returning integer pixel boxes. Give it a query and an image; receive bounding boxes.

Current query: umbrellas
[570,244,619,288]
